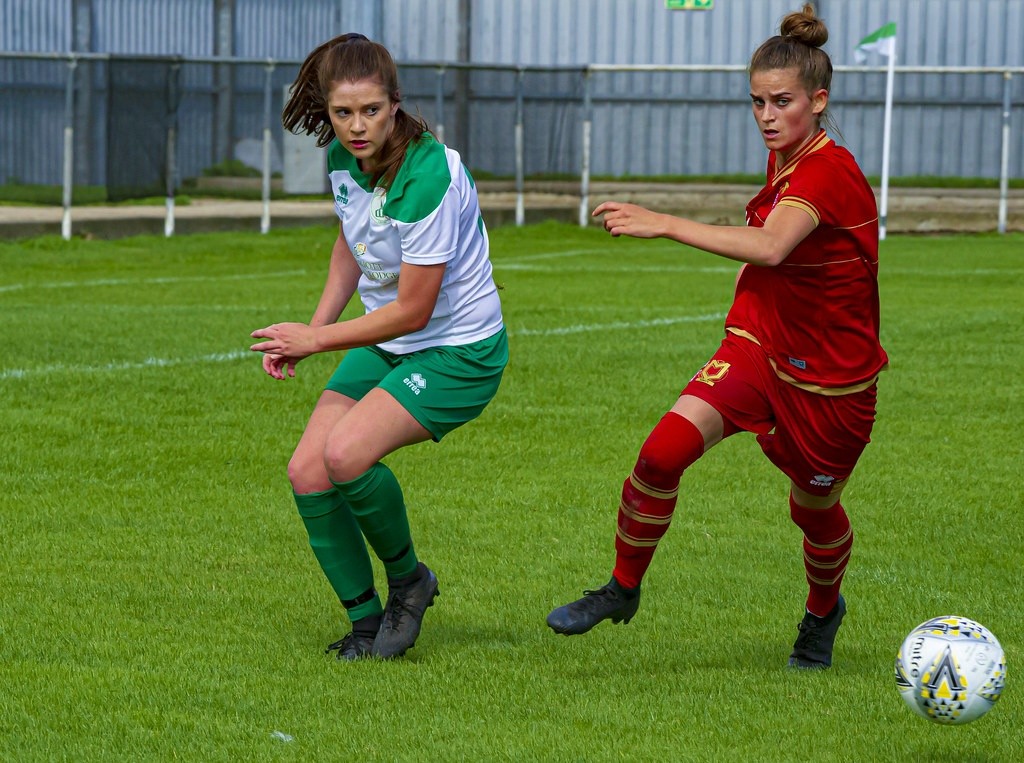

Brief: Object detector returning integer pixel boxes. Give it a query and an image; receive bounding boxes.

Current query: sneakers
[789,594,847,670]
[371,562,440,662]
[548,576,640,637]
[326,632,375,661]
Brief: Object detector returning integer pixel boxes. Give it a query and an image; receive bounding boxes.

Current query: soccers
[894,615,1008,725]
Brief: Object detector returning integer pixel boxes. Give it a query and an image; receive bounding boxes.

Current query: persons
[248,31,511,664]
[545,14,890,673]
